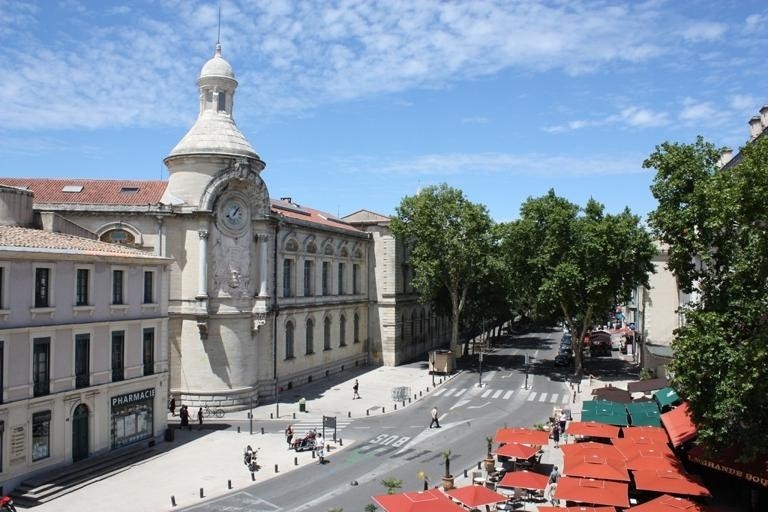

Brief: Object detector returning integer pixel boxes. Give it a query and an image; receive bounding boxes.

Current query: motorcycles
[294,428,316,451]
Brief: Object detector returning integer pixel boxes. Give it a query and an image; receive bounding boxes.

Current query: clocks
[223,202,244,226]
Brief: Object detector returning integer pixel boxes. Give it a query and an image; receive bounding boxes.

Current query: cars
[554,324,591,368]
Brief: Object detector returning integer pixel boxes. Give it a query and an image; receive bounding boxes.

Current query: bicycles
[202,403,225,418]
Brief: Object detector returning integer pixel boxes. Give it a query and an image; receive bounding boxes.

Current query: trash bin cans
[165,429,174,442]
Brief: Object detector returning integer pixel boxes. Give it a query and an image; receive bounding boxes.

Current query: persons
[352,379,361,400]
[169,397,176,415]
[551,418,560,448]
[198,407,204,424]
[544,478,558,506]
[315,432,324,464]
[181,405,190,424]
[179,404,185,421]
[547,465,561,504]
[286,425,294,448]
[245,445,254,462]
[560,410,567,433]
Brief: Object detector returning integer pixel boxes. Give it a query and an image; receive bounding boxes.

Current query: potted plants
[438,449,457,493]
[481,433,497,475]
